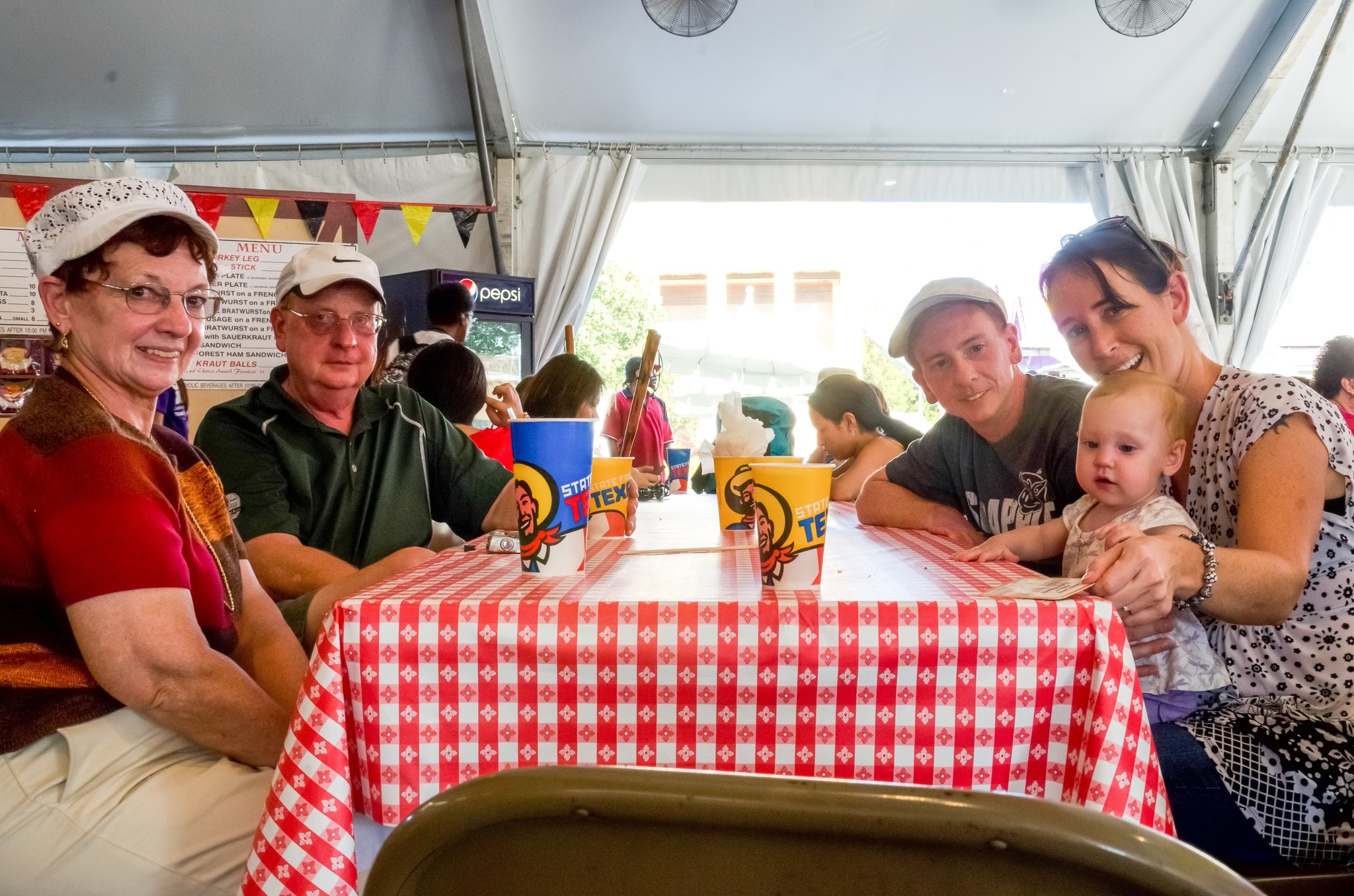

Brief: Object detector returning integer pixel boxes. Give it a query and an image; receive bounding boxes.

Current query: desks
[238,496,1179,896]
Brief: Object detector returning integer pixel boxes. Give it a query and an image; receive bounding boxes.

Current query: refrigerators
[365,268,537,430]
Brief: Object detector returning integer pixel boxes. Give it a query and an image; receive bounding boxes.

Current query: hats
[626,357,661,378]
[888,278,1009,358]
[276,244,388,310]
[24,177,220,282]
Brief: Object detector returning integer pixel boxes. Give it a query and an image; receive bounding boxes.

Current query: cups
[508,419,598,574]
[755,456,804,464]
[747,464,837,589]
[713,457,756,532]
[587,456,634,541]
[667,448,690,494]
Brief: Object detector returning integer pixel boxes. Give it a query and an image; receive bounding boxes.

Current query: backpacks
[378,335,431,385]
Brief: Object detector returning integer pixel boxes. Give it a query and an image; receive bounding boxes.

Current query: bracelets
[1172,532,1219,611]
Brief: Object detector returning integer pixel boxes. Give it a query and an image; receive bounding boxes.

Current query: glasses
[638,487,665,501]
[287,309,387,336]
[84,278,225,319]
[463,312,478,323]
[1075,216,1172,274]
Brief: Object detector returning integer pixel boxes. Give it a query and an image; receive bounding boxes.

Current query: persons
[366,280,674,553]
[1289,335,1354,437]
[805,367,924,501]
[153,378,189,443]
[855,279,1097,578]
[0,175,308,896]
[949,368,1231,722]
[1039,216,1354,877]
[192,243,638,657]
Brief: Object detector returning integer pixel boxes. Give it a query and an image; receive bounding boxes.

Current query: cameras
[486,529,520,553]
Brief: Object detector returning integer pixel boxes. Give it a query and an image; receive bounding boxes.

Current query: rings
[1122,605,1133,615]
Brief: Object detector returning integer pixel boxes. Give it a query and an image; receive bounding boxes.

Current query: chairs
[364,765,1274,896]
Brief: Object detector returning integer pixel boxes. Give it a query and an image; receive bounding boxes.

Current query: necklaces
[80,382,235,613]
